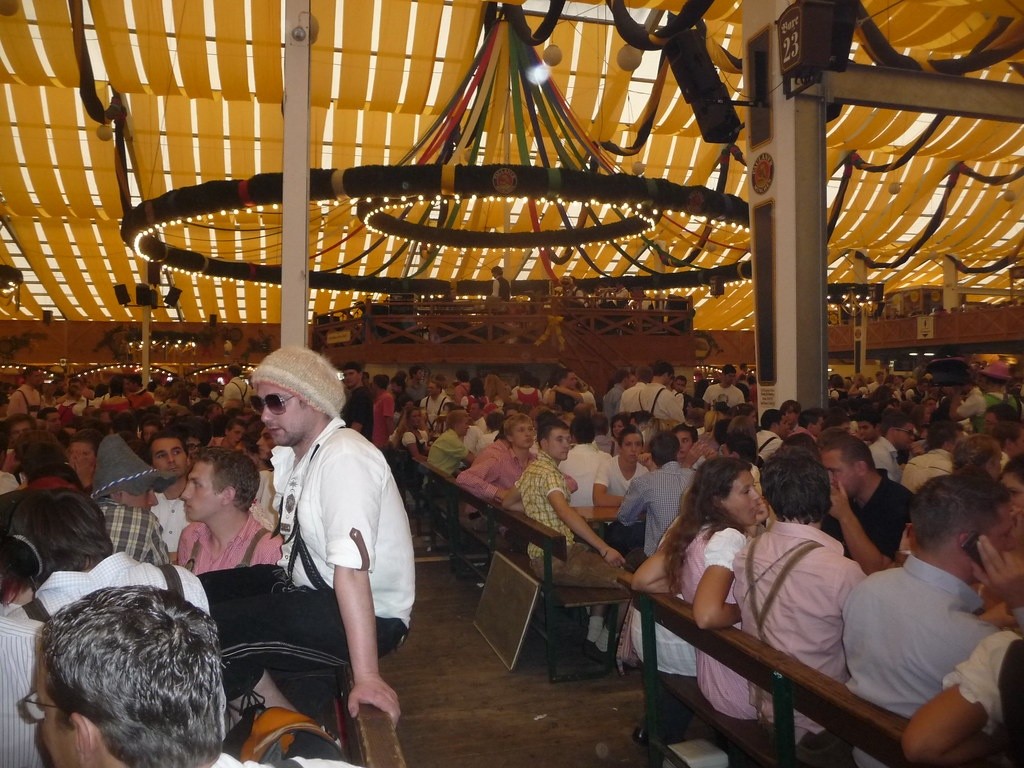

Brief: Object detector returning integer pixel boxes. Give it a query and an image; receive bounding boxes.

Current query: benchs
[412,454,1013,768]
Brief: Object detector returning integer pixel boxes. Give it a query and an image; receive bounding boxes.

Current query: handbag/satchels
[616,597,642,678]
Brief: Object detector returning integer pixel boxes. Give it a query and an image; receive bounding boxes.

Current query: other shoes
[584,640,607,663]
[632,726,649,746]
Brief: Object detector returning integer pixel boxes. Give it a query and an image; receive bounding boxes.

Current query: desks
[572,507,646,540]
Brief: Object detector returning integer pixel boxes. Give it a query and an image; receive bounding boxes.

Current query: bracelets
[602,551,608,559]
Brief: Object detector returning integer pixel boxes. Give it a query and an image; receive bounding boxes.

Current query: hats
[90,433,177,500]
[980,361,1011,381]
[251,346,345,418]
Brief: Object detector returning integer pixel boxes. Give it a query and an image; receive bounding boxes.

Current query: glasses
[23,690,60,720]
[249,393,295,415]
[187,443,202,450]
[895,427,913,435]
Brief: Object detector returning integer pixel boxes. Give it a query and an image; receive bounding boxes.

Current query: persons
[556,275,660,333]
[0,345,1022,768]
[442,287,456,303]
[489,266,511,341]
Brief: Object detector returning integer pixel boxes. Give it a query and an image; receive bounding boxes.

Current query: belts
[468,511,481,520]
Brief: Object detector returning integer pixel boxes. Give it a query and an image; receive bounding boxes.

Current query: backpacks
[222,703,347,763]
